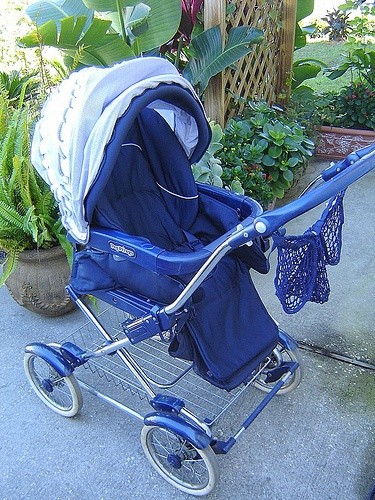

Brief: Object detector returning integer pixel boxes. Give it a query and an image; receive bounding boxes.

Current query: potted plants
[0,44,98,317]
[225,96,315,200]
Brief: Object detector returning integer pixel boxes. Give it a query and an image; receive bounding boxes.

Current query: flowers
[215,120,276,212]
[320,80,375,131]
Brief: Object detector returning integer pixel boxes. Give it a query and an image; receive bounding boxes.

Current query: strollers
[19,55,375,496]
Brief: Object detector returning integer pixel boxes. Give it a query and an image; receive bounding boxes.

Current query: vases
[309,126,375,159]
